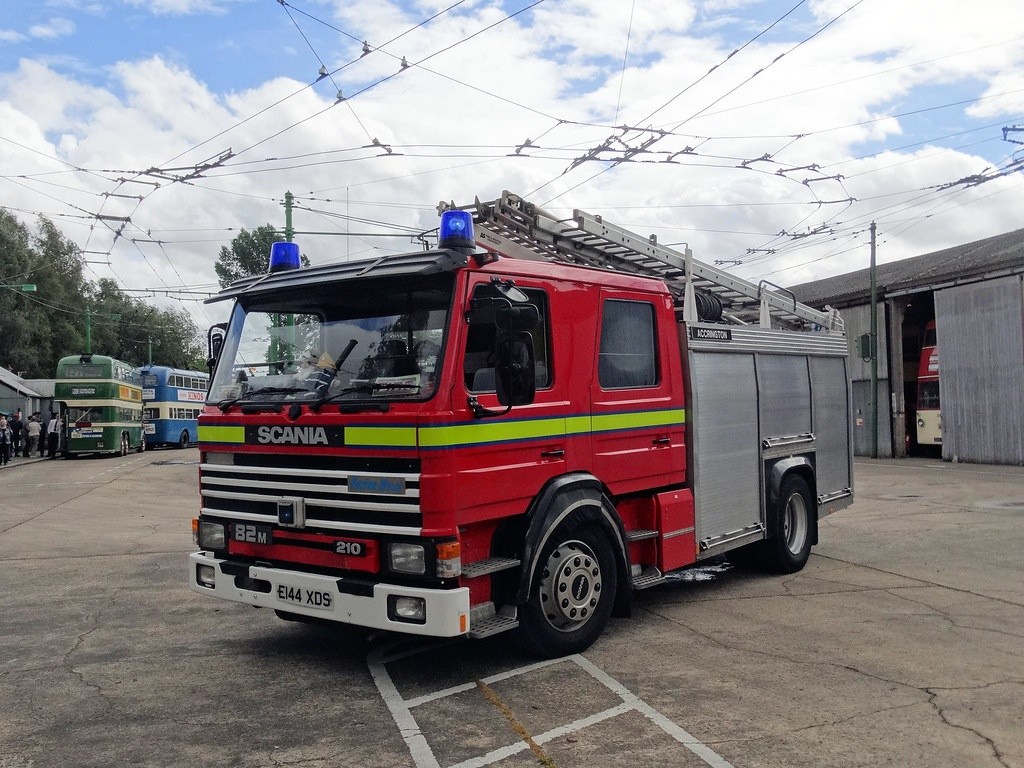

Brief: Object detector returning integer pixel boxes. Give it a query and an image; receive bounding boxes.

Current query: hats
[316,353,338,369]
[415,340,438,372]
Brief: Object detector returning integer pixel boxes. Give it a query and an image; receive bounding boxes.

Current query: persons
[120,409,132,420]
[351,358,374,388]
[0,408,62,465]
[170,410,192,419]
[90,409,102,421]
[290,350,339,392]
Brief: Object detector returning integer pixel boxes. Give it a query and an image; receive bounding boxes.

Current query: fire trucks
[187,188,856,660]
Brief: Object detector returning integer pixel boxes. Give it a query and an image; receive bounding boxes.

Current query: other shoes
[0,454,56,465]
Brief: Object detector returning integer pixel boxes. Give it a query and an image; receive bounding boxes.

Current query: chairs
[371,341,420,374]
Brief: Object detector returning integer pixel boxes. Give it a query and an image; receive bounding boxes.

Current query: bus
[49,352,146,460]
[909,319,941,445]
[136,365,209,451]
